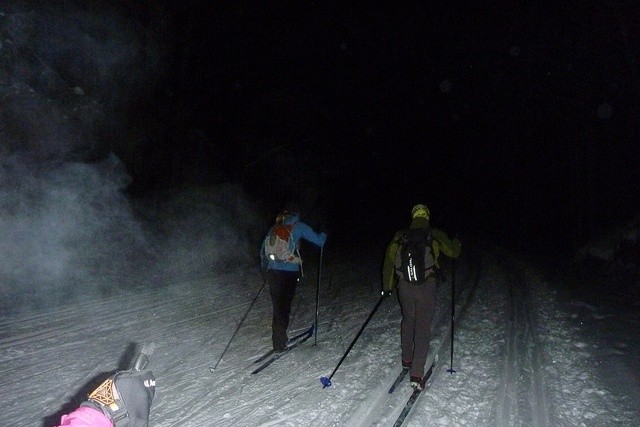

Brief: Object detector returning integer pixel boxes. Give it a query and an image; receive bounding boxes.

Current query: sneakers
[402,360,412,370]
[275,345,288,352]
[410,374,426,390]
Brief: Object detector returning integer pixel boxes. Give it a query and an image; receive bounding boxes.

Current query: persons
[259,201,328,351]
[382,204,462,389]
[62,339,158,427]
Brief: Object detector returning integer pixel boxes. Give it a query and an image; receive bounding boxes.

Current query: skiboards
[388,362,436,426]
[251,324,314,375]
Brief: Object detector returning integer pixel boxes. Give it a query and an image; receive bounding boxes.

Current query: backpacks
[263,220,304,264]
[395,226,441,284]
